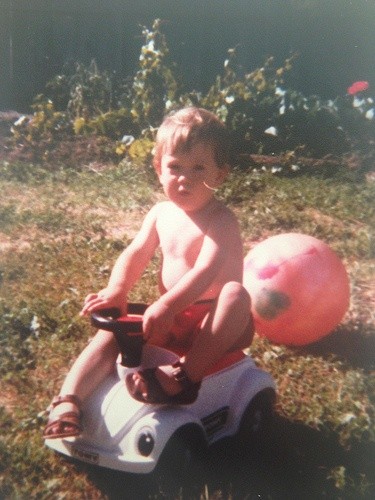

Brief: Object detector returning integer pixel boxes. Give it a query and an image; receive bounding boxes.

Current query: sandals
[126,360,202,404]
[43,394,81,439]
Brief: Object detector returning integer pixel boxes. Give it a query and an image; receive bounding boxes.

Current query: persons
[42,107,254,438]
